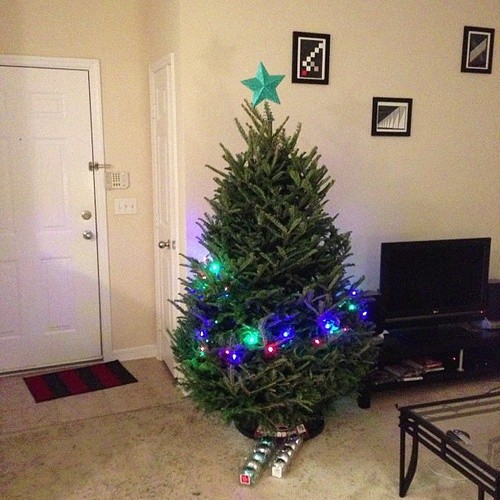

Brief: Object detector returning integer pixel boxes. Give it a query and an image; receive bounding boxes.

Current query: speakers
[361,290,382,324]
[487,279,500,321]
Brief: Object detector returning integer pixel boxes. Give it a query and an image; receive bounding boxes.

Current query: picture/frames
[461,26,495,74]
[371,97,413,136]
[291,31,330,84]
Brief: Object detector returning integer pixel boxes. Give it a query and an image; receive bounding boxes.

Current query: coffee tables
[395,387,500,496]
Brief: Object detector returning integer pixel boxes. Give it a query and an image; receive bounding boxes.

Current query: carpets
[23,360,138,403]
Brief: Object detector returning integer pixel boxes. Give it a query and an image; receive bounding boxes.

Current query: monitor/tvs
[380,237,491,327]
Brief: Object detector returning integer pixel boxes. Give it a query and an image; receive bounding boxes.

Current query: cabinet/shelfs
[350,279,500,409]
